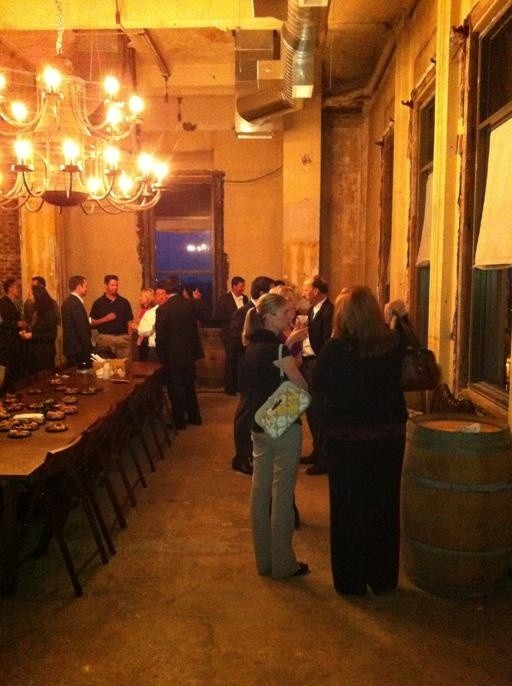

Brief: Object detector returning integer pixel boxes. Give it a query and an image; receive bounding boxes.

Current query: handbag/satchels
[400,344,439,391]
[254,381,313,439]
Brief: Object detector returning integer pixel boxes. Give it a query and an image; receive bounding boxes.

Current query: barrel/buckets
[195,327,227,387]
[399,411,511,604]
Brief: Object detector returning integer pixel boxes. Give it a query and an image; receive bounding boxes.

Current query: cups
[297,316,309,331]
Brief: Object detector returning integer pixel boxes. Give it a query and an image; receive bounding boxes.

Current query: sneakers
[293,562,308,575]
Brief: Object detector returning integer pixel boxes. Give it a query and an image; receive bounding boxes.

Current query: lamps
[0,0,169,215]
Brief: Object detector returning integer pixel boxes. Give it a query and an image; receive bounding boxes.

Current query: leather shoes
[166,417,200,430]
[232,458,253,474]
[300,455,319,464]
[307,463,329,475]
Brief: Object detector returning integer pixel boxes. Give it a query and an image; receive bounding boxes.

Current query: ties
[310,309,314,323]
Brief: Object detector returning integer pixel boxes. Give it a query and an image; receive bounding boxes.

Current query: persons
[216,277,353,526]
[0,276,209,429]
[239,293,309,579]
[309,285,408,596]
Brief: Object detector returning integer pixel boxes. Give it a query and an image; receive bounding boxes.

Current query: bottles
[102,363,111,381]
